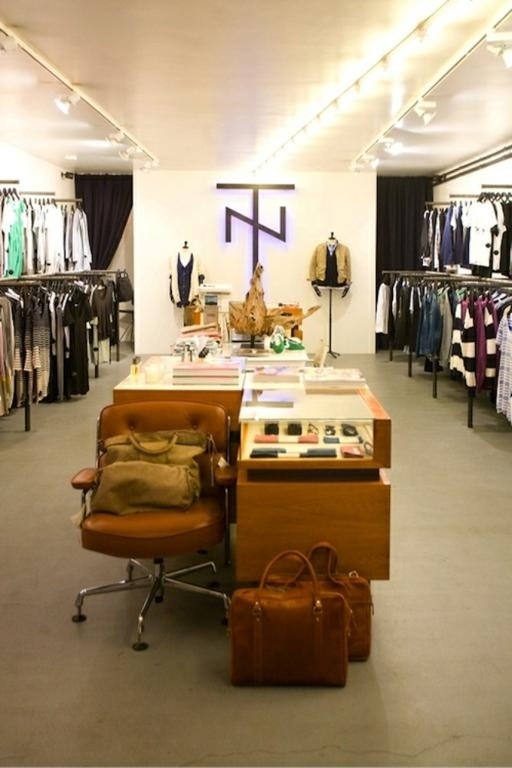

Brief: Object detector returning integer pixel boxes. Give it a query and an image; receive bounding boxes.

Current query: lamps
[0,22,159,171]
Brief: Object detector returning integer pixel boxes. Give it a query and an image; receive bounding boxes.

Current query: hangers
[0,271,113,294]
[0,186,86,212]
[382,274,512,298]
[424,191,512,216]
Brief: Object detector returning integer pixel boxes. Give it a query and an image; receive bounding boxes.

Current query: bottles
[132,355,141,381]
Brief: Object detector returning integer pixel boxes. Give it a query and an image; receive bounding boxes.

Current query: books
[173,357,244,376]
[173,376,243,385]
[252,366,301,383]
[305,390,359,395]
[304,367,367,390]
[240,388,294,408]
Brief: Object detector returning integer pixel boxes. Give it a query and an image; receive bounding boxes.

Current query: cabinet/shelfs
[195,277,232,339]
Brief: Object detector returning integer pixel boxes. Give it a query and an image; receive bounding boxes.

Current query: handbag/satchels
[231,550,351,687]
[266,542,374,661]
[91,430,208,515]
[117,271,133,302]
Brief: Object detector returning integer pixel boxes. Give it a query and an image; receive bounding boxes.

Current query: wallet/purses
[251,448,278,458]
[301,449,337,457]
[298,435,318,443]
[264,424,279,435]
[324,437,339,443]
[288,424,302,435]
[341,446,364,457]
[255,434,279,443]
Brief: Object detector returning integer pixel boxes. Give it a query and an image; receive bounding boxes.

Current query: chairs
[69,396,236,650]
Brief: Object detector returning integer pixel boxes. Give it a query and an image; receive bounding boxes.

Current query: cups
[222,341,232,360]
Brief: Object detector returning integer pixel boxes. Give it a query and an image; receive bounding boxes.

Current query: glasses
[308,423,319,434]
[341,424,358,436]
[324,425,336,435]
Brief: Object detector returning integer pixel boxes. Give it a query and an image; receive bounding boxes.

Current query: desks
[111,348,395,584]
